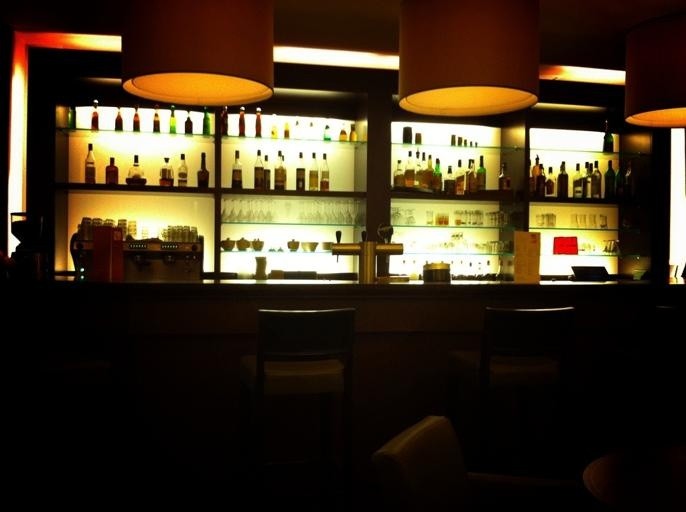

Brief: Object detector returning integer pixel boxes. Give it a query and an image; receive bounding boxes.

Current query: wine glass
[220,196,360,226]
[601,239,622,255]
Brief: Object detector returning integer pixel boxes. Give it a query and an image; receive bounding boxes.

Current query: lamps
[114,0,278,109]
[394,1,543,116]
[623,0,686,132]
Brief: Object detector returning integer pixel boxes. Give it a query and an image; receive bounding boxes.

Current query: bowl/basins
[221,237,334,251]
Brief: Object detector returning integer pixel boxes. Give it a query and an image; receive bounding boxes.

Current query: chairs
[471,300,581,442]
[242,301,361,486]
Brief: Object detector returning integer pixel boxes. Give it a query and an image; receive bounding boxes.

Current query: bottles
[85,144,330,193]
[399,259,506,280]
[393,150,638,201]
[602,119,614,153]
[66,99,357,144]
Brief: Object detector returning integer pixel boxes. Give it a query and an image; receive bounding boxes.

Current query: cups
[403,127,412,144]
[536,213,609,230]
[451,135,477,147]
[79,216,198,242]
[336,231,342,244]
[392,206,511,227]
[416,133,421,144]
[436,230,509,253]
[361,230,368,242]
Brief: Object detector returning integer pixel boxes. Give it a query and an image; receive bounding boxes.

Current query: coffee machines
[77,238,207,281]
[9,212,45,280]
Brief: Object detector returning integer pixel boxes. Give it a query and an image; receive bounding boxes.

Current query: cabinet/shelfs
[54,102,219,274]
[369,109,526,275]
[522,112,669,279]
[214,100,368,275]
